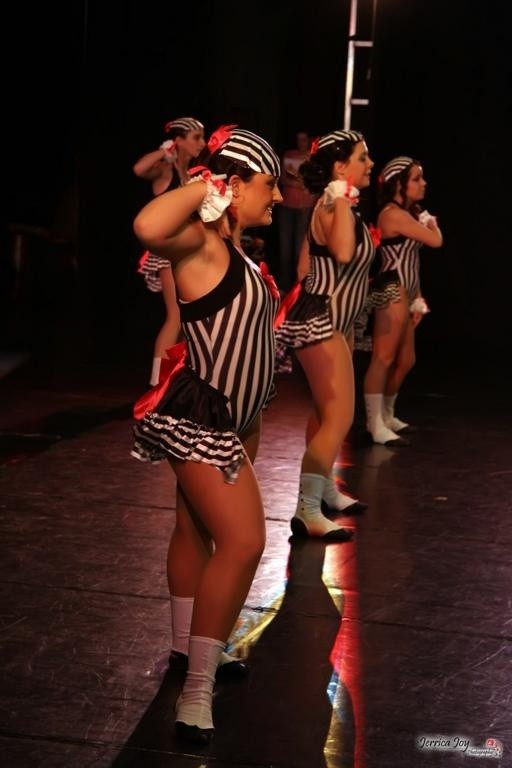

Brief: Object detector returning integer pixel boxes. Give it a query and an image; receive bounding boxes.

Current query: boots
[169,593,248,686]
[360,393,417,448]
[174,636,226,749]
[290,473,368,542]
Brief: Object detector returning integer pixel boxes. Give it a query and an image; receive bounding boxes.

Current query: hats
[378,155,414,185]
[309,129,365,155]
[164,117,205,134]
[207,124,282,178]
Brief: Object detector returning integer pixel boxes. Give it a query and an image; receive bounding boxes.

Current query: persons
[134,118,207,386]
[273,131,377,541]
[132,124,283,754]
[280,129,307,273]
[363,156,444,446]
